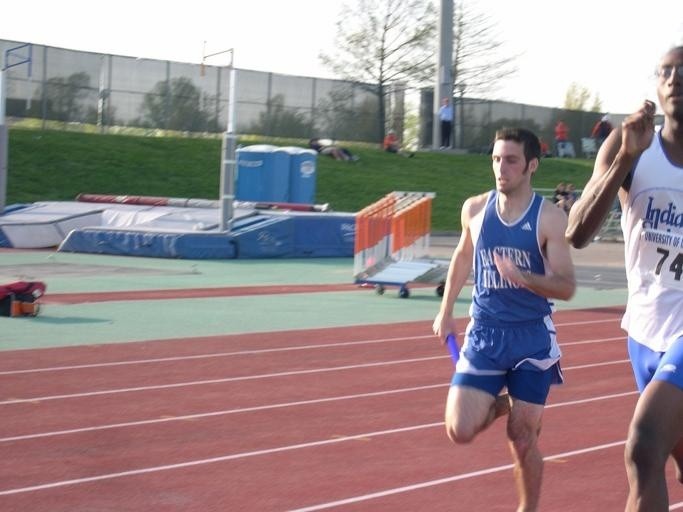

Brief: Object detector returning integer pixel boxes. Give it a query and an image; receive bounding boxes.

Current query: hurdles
[351,190,448,301]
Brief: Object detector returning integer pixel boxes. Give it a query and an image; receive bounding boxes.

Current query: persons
[309,137,351,162]
[535,115,614,215]
[382,129,415,159]
[432,125,577,511]
[565,48,683,512]
[439,96,454,150]
